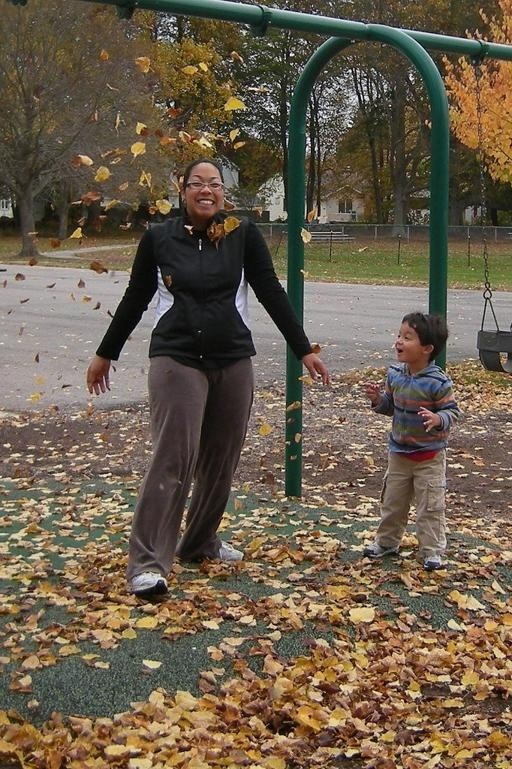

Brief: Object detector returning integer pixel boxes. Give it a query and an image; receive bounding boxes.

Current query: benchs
[309,230,357,243]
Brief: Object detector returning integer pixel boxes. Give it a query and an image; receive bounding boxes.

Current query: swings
[476,64,510,373]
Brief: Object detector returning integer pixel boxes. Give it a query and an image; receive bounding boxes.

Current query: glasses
[185,182,224,190]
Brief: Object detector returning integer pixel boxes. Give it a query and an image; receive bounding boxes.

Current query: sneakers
[126,572,167,595]
[423,554,442,570]
[214,541,244,561]
[362,542,399,558]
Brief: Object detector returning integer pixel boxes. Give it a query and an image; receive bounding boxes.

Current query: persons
[362,311,461,572]
[86,157,332,597]
